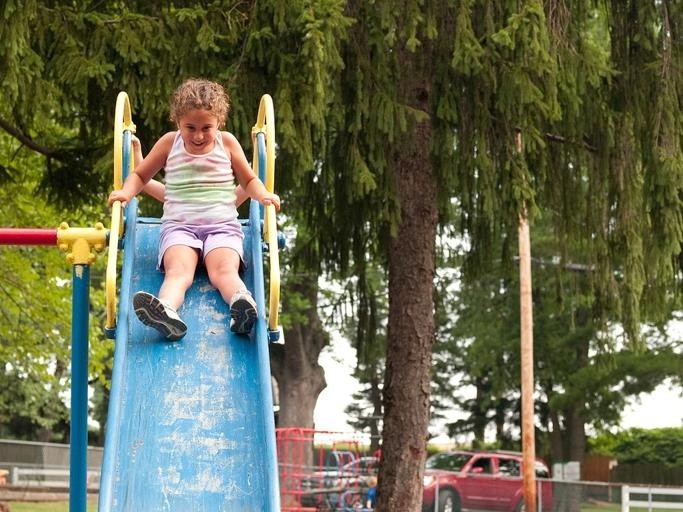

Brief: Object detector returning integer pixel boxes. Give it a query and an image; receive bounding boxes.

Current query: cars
[422,451,553,512]
[295,457,380,507]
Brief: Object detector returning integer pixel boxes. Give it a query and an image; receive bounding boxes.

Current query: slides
[98,194,281,511]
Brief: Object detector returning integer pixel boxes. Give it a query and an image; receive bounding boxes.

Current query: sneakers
[230,292,258,334]
[133,290,187,341]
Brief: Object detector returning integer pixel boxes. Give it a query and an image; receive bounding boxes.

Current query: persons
[131,133,249,210]
[365,478,377,508]
[107,74,283,342]
